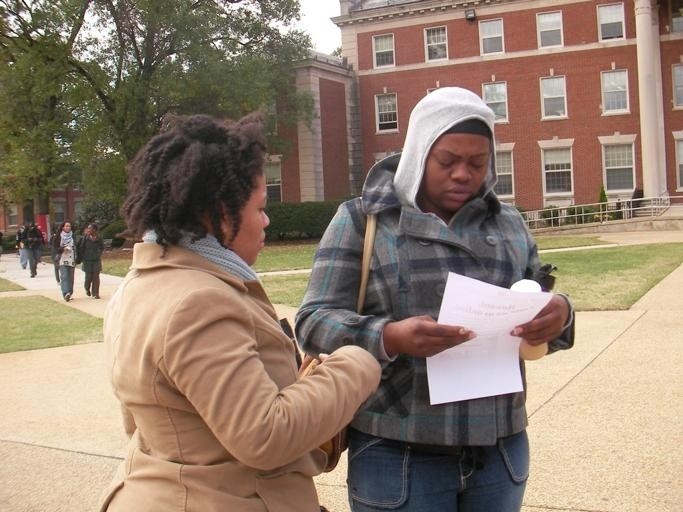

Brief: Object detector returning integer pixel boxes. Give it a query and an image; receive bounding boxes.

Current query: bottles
[519,263,559,361]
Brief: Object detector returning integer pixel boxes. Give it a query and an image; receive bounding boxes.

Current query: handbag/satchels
[76,238,85,264]
[54,252,62,263]
[298,353,349,474]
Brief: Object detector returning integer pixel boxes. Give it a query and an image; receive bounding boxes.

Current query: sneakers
[85,289,101,299]
[22,264,39,278]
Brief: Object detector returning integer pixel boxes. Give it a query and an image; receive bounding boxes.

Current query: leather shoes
[65,293,71,303]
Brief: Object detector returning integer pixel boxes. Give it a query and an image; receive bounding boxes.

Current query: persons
[294,86,576,512]
[96,109,383,512]
[16,221,103,302]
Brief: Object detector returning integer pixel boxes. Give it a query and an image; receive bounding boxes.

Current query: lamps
[464,9,476,22]
